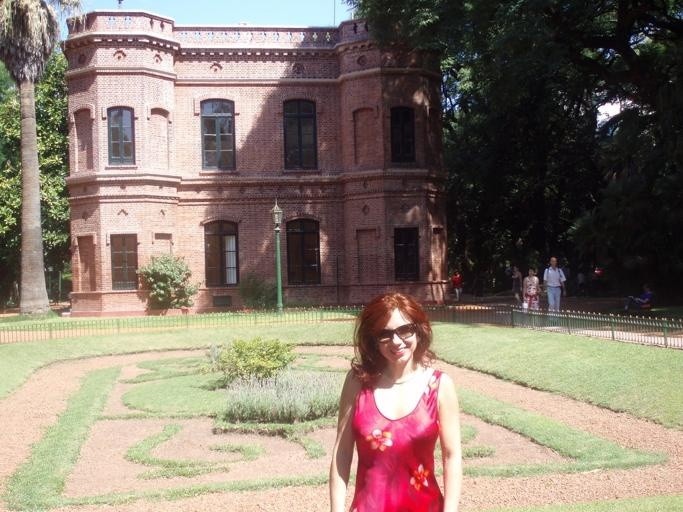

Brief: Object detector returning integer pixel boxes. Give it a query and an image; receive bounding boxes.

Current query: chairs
[639,293,658,313]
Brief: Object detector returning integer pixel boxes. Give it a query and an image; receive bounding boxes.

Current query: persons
[522,266,540,328]
[451,268,465,302]
[623,283,653,313]
[511,263,523,302]
[542,256,566,327]
[328,291,463,512]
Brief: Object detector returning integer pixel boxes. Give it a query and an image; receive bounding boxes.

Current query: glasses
[374,322,417,344]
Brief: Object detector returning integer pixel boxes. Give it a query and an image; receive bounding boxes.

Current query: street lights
[268,196,290,316]
